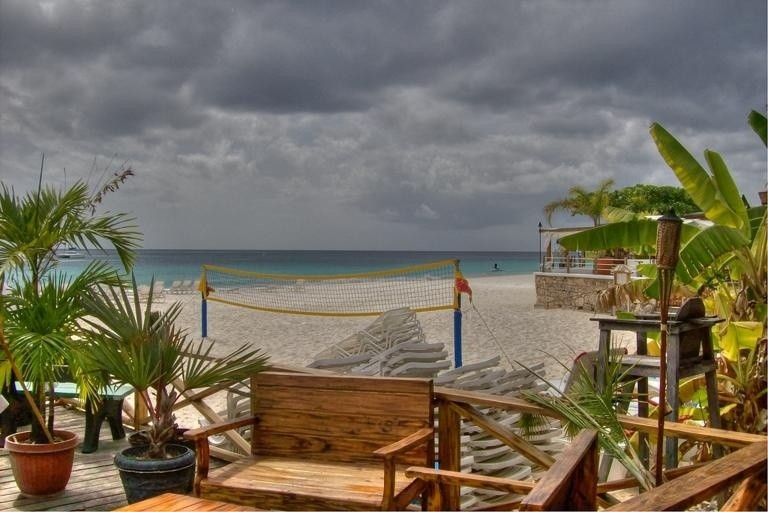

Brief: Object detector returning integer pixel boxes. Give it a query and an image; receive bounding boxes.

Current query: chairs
[211,308,451,462]
[587,297,729,492]
[36,277,205,304]
[422,354,564,507]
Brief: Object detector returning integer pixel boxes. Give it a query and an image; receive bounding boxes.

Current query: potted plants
[61,275,270,505]
[2,150,148,500]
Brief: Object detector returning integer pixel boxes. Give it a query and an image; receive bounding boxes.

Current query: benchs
[8,380,139,453]
[186,370,442,510]
[406,429,609,509]
